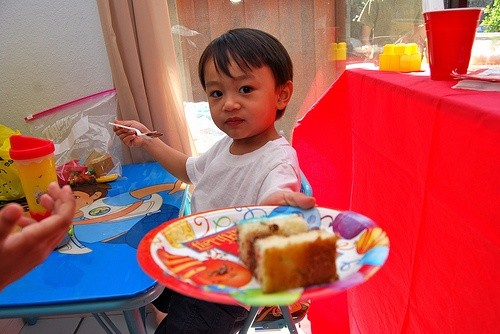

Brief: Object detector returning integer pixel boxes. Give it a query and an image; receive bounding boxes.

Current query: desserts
[237,214,338,294]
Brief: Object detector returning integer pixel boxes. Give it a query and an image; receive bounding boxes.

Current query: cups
[9,134,60,222]
[422,7,483,80]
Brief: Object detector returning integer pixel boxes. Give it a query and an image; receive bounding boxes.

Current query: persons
[0,181,77,290]
[112,27,322,334]
[353,0,428,64]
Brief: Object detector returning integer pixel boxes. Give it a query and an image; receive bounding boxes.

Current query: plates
[135,203,390,308]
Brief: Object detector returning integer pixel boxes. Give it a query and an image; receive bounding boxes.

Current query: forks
[108,122,163,139]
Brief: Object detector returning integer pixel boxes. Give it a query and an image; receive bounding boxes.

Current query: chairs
[239,165,313,334]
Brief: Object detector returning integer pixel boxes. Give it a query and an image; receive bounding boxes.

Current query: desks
[0,161,189,334]
[292,64,500,334]
